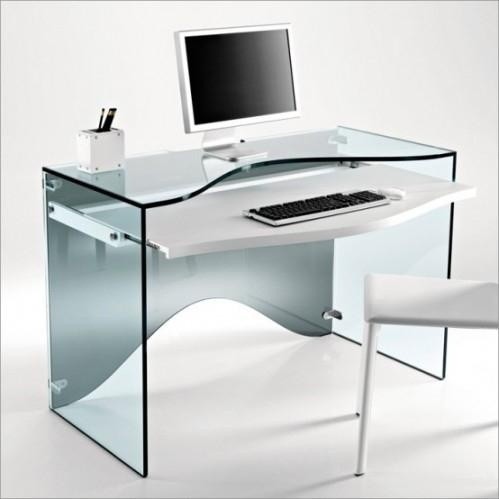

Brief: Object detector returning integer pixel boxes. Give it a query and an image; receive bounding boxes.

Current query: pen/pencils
[98,108,116,131]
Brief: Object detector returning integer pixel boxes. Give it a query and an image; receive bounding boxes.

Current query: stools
[353,273,499,477]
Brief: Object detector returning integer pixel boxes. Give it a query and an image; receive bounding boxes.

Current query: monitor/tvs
[174,22,302,162]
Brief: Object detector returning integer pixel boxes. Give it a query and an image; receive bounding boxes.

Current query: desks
[42,126,477,477]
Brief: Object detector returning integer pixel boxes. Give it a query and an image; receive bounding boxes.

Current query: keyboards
[241,187,389,227]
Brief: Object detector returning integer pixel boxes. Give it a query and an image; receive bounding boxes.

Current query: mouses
[378,187,403,199]
